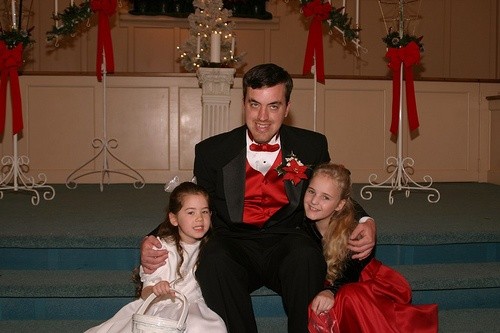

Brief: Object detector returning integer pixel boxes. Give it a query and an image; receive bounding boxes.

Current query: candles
[231,38,235,56]
[211,34,221,63]
[197,36,200,54]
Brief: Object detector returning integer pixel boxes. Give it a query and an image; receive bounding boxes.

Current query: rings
[324,310,329,313]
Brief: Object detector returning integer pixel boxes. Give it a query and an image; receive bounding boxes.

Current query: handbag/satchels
[132,288,189,333]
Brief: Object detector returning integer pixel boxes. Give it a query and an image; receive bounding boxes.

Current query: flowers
[44,2,92,41]
[0,24,36,48]
[382,27,425,53]
[302,0,361,41]
[274,151,308,185]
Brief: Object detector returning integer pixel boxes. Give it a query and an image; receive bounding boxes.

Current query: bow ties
[248,129,280,152]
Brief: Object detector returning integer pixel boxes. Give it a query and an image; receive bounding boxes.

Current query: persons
[141,64,375,332]
[83,182,228,332]
[304,163,438,332]
